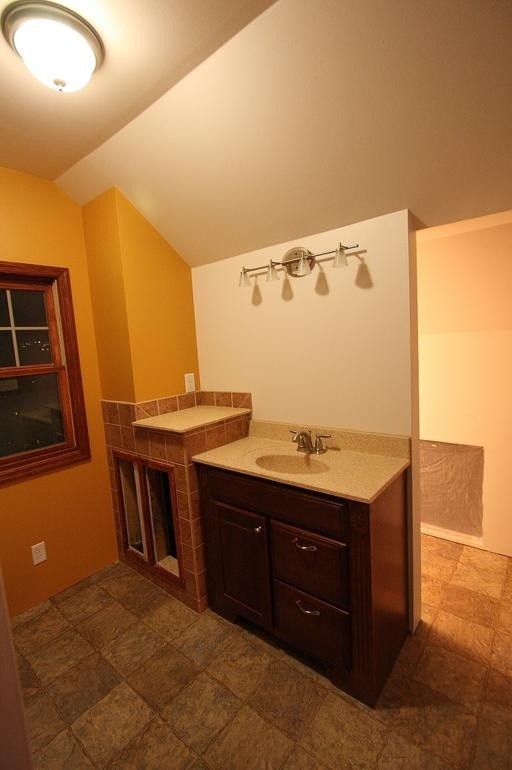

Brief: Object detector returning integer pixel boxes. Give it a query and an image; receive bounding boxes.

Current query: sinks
[256,454,329,475]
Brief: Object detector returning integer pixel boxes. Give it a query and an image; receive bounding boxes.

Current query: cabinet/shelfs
[198,470,408,710]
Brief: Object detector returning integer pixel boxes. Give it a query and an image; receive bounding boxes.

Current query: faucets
[292,431,313,450]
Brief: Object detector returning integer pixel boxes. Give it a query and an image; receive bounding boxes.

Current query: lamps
[238,240,349,289]
